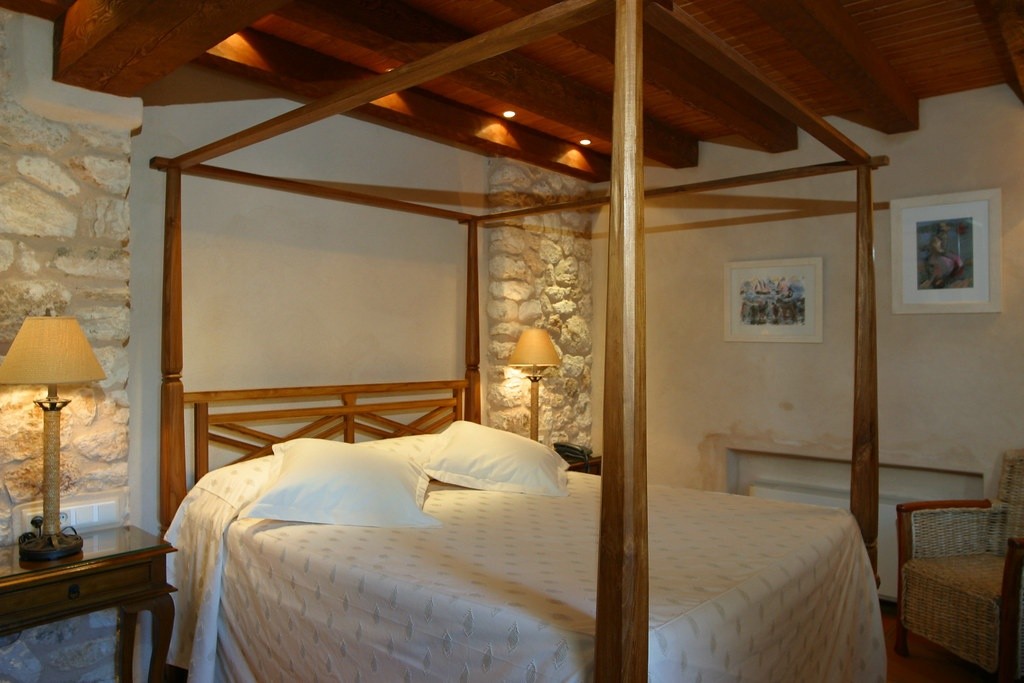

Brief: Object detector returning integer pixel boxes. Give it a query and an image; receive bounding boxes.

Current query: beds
[148,0,889,683]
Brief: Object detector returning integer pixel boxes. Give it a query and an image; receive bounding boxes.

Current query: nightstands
[558,453,603,477]
[2,524,180,683]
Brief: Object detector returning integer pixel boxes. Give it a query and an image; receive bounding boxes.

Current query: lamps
[0,316,108,561]
[509,327,562,443]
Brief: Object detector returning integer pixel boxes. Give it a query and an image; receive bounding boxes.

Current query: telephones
[553,442,593,461]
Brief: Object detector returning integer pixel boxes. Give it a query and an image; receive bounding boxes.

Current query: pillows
[423,420,571,498]
[237,438,446,536]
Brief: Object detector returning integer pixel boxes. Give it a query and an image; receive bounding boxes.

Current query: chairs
[895,447,1024,683]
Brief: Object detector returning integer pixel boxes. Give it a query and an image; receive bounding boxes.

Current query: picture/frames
[722,257,824,344]
[889,187,1004,316]
